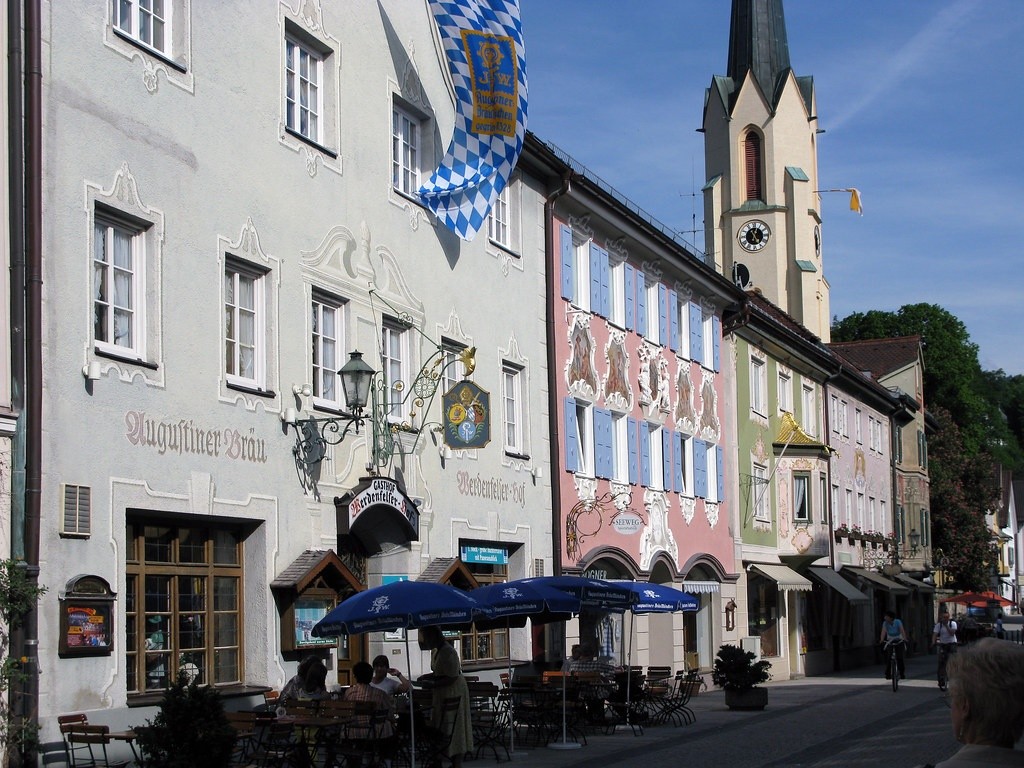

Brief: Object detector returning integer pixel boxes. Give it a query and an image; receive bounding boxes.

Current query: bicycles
[881,638,908,692]
[930,642,961,690]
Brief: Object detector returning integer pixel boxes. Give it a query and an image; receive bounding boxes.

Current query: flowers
[851,523,883,537]
[887,532,897,541]
[649,680,673,692]
[837,523,850,532]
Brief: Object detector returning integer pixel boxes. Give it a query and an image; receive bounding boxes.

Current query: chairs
[58,665,699,768]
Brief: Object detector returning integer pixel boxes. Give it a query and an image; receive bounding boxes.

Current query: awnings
[1003,579,1015,585]
[842,566,934,594]
[807,566,869,605]
[750,564,812,591]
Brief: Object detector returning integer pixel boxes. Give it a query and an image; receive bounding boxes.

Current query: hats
[886,609,896,618]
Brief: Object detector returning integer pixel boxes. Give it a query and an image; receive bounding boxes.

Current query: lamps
[83,361,101,380]
[531,467,542,477]
[440,447,451,459]
[279,348,376,464]
[897,529,920,556]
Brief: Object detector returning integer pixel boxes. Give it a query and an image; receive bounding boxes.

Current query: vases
[835,531,848,537]
[646,686,667,693]
[885,539,900,546]
[849,533,883,543]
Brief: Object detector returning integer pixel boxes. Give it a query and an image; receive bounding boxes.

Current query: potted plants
[682,674,707,697]
[712,645,773,710]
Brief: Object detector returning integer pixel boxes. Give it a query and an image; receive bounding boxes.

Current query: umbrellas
[937,589,1018,617]
[311,579,496,768]
[612,581,699,725]
[471,581,583,754]
[526,577,640,744]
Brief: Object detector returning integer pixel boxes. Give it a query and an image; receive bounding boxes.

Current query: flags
[413,0,528,242]
[847,188,862,213]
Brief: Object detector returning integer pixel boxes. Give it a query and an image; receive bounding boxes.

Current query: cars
[967,602,1003,618]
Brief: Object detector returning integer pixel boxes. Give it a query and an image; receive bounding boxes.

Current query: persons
[511,643,649,725]
[936,637,1024,768]
[149,616,163,642]
[79,632,106,646]
[278,655,413,768]
[995,613,1006,640]
[1019,598,1024,616]
[932,612,957,686]
[417,626,473,768]
[880,610,906,680]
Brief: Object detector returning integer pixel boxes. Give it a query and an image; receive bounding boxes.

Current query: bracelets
[397,673,401,677]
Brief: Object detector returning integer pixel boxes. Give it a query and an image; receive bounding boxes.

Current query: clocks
[740,222,769,251]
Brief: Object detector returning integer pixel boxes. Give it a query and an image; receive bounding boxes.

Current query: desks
[506,686,569,745]
[582,682,617,736]
[644,676,673,724]
[105,730,257,768]
[258,716,344,768]
[391,704,434,768]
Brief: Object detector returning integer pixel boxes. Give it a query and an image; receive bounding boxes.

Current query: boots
[428,757,443,768]
[448,753,462,768]
